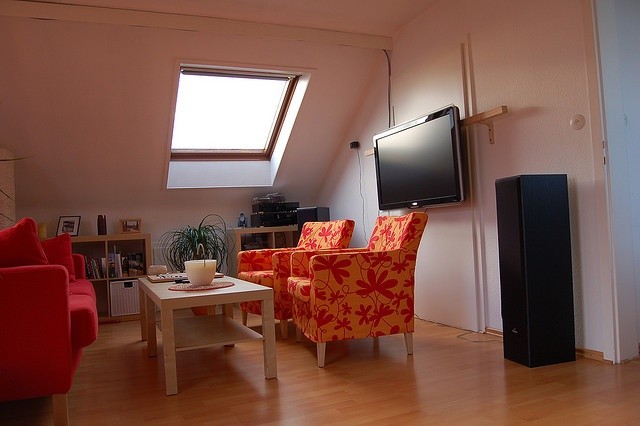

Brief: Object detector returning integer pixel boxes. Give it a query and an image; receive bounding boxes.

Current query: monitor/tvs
[373,103,467,210]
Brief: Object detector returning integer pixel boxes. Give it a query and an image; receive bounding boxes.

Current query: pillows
[0,217,47,265]
[41,232,76,281]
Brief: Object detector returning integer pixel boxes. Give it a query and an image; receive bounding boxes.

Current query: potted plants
[156,214,237,273]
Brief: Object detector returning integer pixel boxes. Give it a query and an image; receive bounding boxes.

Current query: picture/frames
[56,216,81,236]
[117,216,143,234]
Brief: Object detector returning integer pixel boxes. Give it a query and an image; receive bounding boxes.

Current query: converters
[350,140,360,149]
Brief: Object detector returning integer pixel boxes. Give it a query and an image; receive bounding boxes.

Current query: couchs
[288,213,429,369]
[237,219,355,341]
[1,253,100,426]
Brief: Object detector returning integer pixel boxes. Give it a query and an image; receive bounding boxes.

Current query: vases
[184,259,217,286]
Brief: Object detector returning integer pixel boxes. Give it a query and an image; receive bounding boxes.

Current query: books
[85,256,107,279]
[108,245,122,277]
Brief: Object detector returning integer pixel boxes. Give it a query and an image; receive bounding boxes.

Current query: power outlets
[348,139,360,152]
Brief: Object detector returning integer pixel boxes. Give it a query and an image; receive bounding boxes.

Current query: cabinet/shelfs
[107,238,151,321]
[70,236,109,323]
[274,227,299,248]
[227,224,274,309]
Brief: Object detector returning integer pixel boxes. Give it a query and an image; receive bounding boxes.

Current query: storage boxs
[110,279,140,316]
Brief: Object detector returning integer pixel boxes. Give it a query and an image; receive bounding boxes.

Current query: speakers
[495,173,576,369]
[296,206,330,244]
